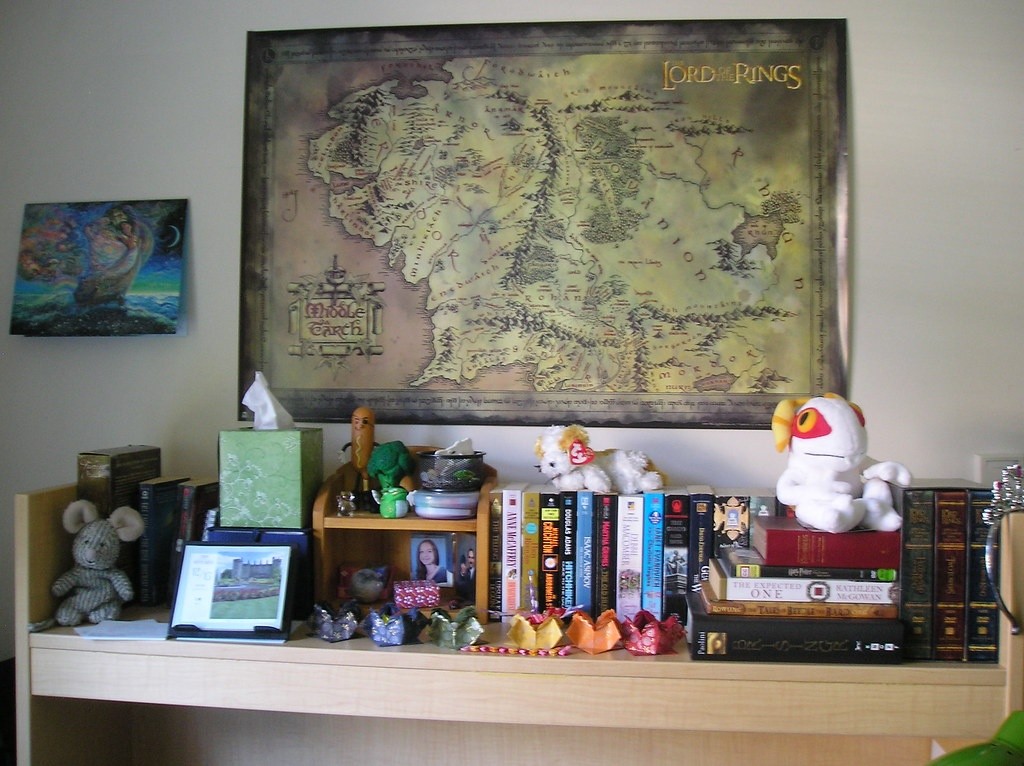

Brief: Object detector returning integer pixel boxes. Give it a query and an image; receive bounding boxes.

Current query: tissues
[217,372,323,530]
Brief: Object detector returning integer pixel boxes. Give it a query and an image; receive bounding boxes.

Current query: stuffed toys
[771,393,913,535]
[50,500,145,627]
[533,424,670,494]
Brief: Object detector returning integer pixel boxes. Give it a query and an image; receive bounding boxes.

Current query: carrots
[350,407,373,473]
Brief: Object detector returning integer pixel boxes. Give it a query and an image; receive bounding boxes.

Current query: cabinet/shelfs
[12,446,1024,766]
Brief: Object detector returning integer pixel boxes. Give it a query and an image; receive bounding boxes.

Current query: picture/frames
[410,532,455,589]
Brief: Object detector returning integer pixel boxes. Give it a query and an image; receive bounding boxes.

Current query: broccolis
[366,440,413,490]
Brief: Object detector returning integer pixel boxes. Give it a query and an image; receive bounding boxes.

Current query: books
[75,444,220,611]
[488,482,1001,666]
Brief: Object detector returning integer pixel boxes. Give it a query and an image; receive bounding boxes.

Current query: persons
[456,549,476,597]
[415,539,447,583]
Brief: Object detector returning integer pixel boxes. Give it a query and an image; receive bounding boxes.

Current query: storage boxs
[216,426,323,530]
[208,527,312,621]
[77,447,161,609]
